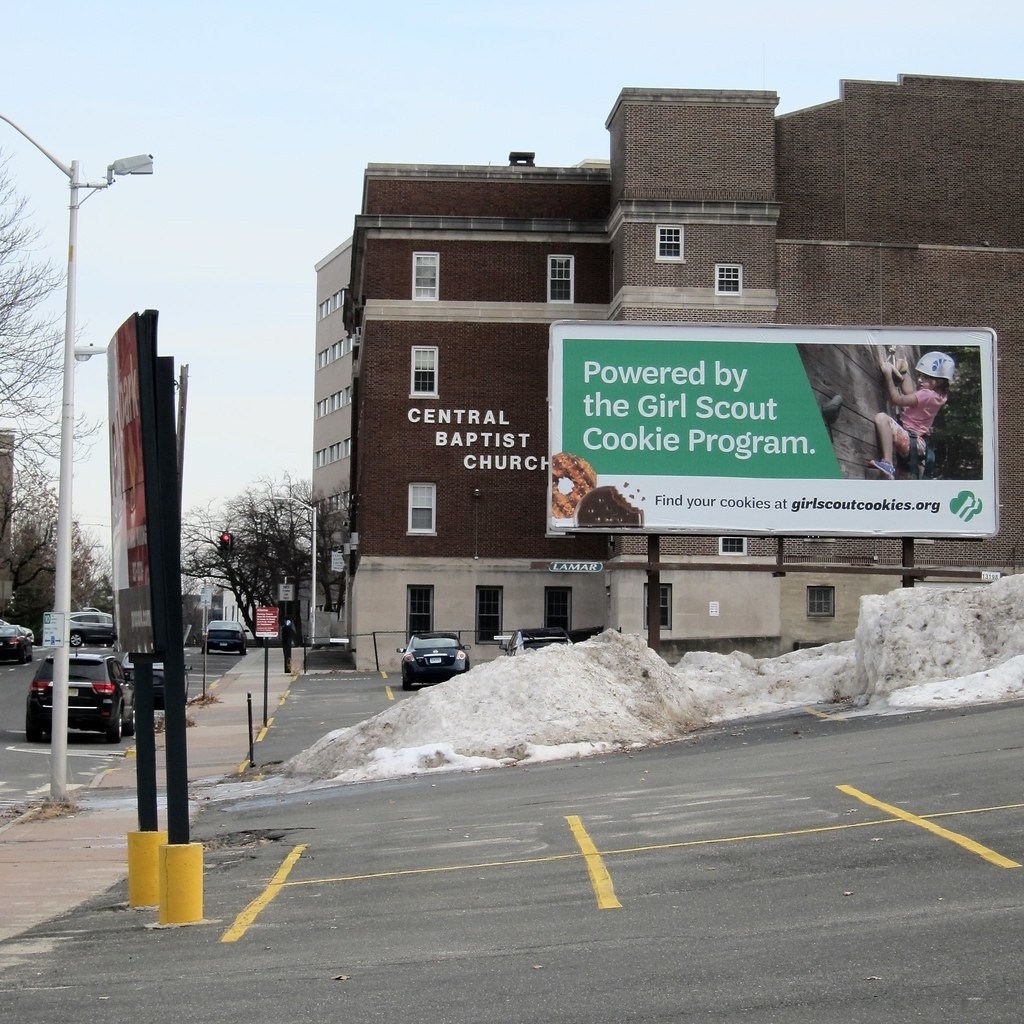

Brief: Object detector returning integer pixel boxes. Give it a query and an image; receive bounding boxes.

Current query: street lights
[274,497,318,648]
[49,149,157,799]
[75,343,189,521]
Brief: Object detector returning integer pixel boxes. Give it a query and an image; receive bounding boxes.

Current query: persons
[869,351,955,480]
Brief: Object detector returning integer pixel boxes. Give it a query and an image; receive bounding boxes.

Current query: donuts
[552,452,597,518]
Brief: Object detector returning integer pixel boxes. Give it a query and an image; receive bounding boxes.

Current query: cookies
[574,485,644,527]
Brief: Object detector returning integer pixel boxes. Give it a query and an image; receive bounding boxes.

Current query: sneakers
[869,459,895,479]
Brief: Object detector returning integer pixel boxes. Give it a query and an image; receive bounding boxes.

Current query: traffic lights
[216,534,224,557]
[230,533,236,551]
[223,534,230,550]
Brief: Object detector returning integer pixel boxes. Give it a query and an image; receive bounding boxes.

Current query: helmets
[914,351,955,381]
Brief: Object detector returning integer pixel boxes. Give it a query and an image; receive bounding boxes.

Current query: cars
[202,620,250,656]
[121,652,193,708]
[396,632,471,690]
[498,627,572,656]
[71,612,116,649]
[0,624,33,663]
[0,620,35,646]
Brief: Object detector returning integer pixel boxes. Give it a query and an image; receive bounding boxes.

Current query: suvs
[26,648,135,743]
